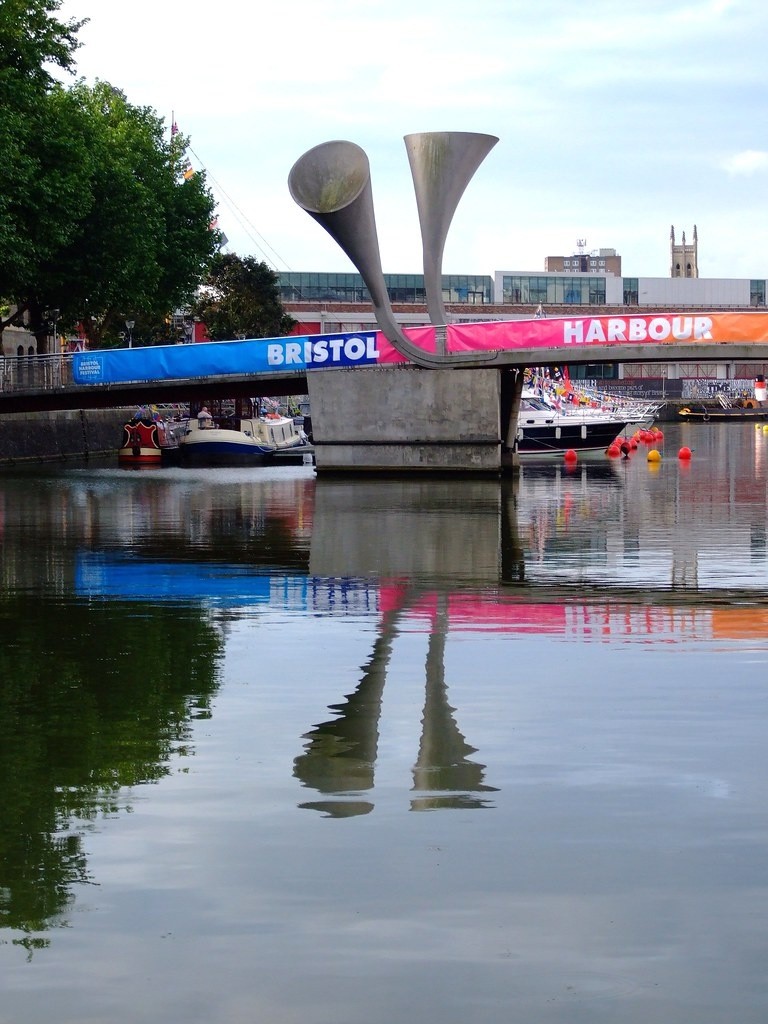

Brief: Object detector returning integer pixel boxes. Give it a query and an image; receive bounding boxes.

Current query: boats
[676,404,768,421]
[513,366,667,454]
[118,396,310,466]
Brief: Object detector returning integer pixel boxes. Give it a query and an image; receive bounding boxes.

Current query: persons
[553,367,563,381]
[732,398,743,408]
[197,407,212,418]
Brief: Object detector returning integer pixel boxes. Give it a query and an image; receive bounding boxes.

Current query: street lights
[125,318,135,348]
[185,323,192,343]
[51,309,60,354]
